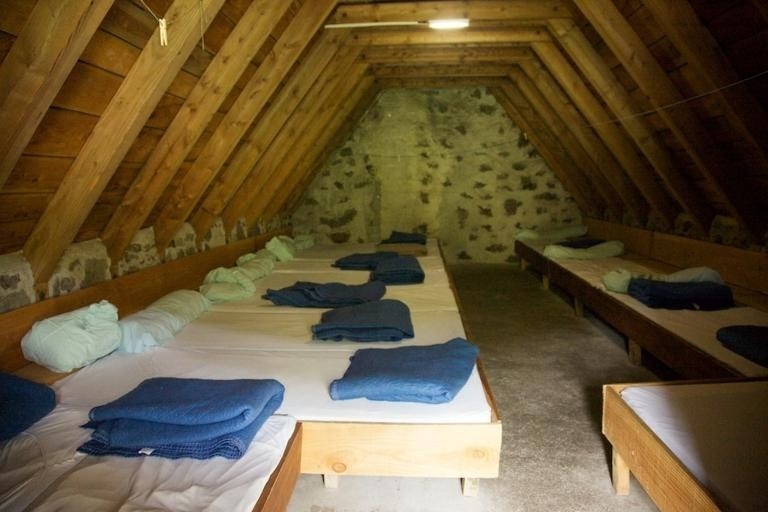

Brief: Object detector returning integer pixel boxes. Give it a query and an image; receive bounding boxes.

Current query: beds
[0,217,503,495]
[0,414,304,512]
[601,378,766,511]
[513,217,768,383]
